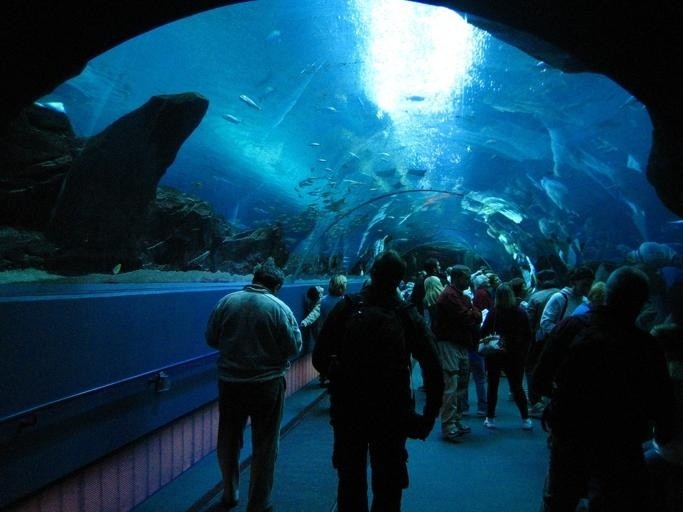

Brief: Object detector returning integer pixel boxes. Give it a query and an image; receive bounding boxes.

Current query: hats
[374,249,406,276]
[537,269,555,280]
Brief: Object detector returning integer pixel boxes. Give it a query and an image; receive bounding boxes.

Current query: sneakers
[443,409,496,437]
[522,417,534,429]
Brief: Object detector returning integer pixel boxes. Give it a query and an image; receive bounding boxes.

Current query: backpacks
[345,295,412,414]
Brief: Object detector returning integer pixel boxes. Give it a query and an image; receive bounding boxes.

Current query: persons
[304,274,347,388]
[312,253,445,512]
[531,265,676,512]
[204,264,303,512]
[393,256,608,442]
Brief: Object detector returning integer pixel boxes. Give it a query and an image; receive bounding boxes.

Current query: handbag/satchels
[477,335,505,355]
[536,326,547,342]
